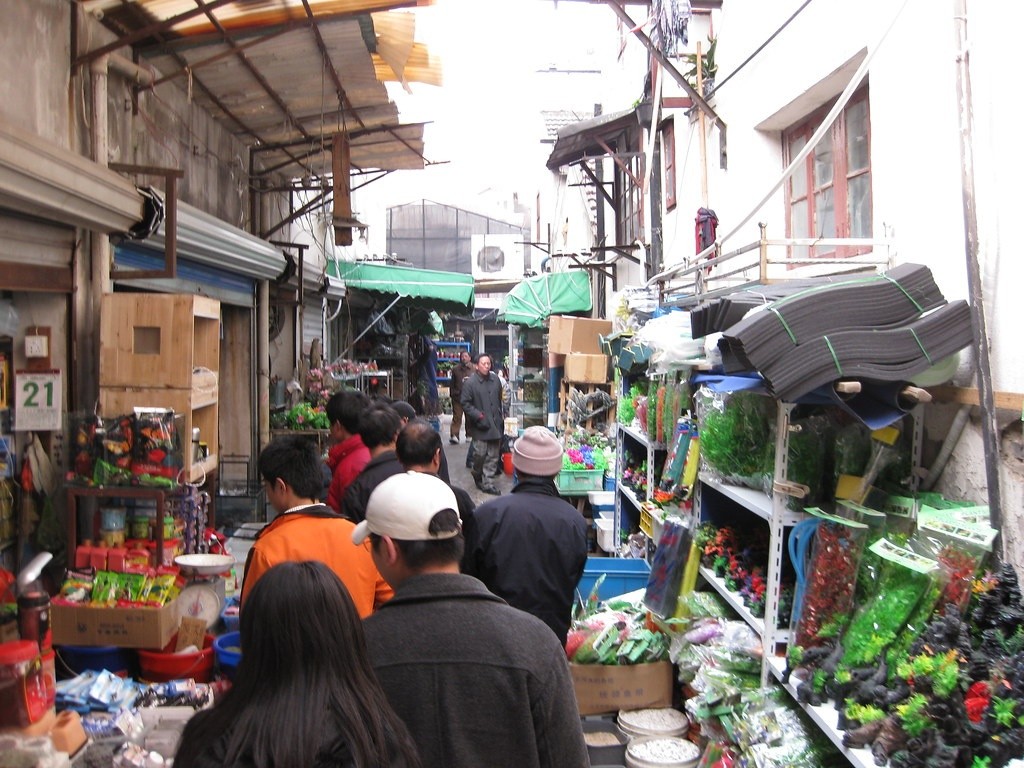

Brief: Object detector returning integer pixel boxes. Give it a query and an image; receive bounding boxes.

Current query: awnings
[546,104,640,169]
[327,260,476,370]
[85,0,442,185]
[496,268,592,328]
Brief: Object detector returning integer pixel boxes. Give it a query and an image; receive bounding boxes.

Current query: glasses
[255,481,270,499]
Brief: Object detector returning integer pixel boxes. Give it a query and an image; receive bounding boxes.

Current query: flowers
[269,401,332,430]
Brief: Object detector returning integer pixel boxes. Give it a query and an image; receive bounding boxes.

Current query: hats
[352,470,463,540]
[512,426,564,476]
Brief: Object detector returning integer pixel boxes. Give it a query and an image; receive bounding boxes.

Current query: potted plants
[679,32,715,108]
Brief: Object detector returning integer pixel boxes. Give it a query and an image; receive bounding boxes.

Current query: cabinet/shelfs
[97,292,220,483]
[65,468,217,572]
[334,367,394,402]
[614,371,1024,768]
[435,342,471,380]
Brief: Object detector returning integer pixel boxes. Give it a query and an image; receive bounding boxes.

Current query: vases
[269,427,330,459]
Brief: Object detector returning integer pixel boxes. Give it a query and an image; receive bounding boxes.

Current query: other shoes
[466,462,475,468]
[466,436,472,442]
[483,485,501,495]
[475,479,483,490]
[449,436,458,443]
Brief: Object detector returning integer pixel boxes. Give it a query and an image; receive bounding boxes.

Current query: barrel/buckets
[616,705,701,768]
[54,630,241,686]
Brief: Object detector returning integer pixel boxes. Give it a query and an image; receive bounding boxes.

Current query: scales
[174,554,235,634]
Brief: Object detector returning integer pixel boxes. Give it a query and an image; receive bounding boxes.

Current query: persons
[460,425,589,656]
[238,435,396,619]
[172,558,420,768]
[460,353,511,496]
[449,352,475,445]
[323,390,475,524]
[351,470,593,768]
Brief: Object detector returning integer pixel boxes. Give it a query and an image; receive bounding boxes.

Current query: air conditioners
[472,234,524,281]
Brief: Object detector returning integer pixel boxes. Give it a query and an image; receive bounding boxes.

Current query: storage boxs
[567,654,674,718]
[215,485,266,538]
[547,313,612,384]
[556,468,680,603]
[220,593,241,633]
[50,593,182,650]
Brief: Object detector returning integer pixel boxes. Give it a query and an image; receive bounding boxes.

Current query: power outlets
[24,336,48,357]
